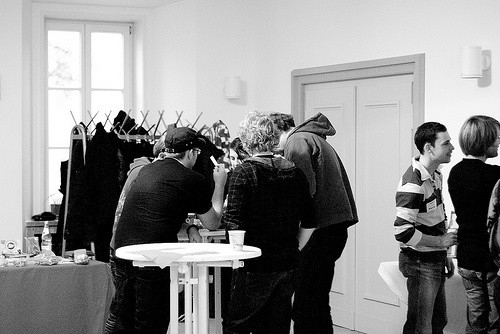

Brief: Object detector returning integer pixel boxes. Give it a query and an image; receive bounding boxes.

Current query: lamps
[224,71,241,99]
[459,46,491,80]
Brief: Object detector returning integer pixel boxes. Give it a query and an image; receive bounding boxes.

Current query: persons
[103,109,358,334]
[448,116,500,334]
[392,121,458,334]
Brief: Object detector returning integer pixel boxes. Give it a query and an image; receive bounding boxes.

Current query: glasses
[192,148,201,154]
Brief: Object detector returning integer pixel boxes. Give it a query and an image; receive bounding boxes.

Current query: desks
[25,216,57,256]
[114,241,262,333]
[0,258,115,334]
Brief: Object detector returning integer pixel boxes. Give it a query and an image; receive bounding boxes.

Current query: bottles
[41,221,51,254]
[446,211,457,259]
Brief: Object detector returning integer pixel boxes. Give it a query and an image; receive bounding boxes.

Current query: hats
[165,128,206,153]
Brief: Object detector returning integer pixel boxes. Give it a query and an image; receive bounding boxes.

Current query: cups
[228,230,246,252]
[25,236,40,257]
[74,249,86,265]
